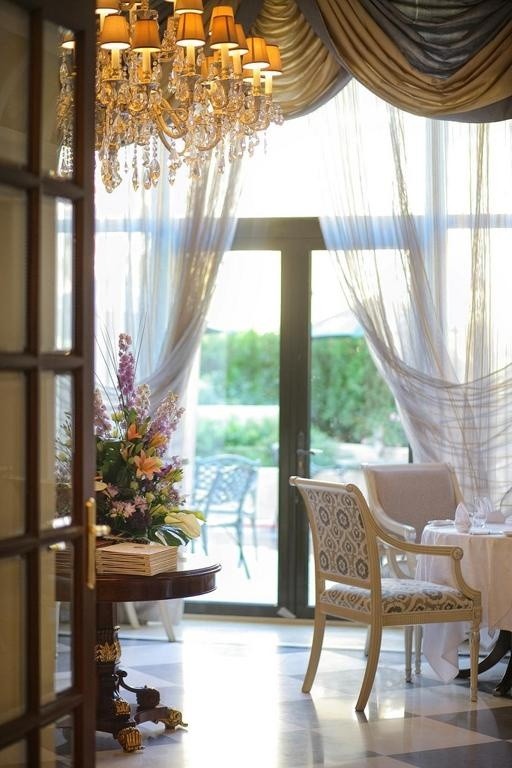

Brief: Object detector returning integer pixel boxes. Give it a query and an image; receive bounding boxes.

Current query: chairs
[361,462,467,674]
[188,455,261,578]
[289,476,483,712]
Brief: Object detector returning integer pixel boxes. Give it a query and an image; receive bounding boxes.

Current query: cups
[471,497,487,529]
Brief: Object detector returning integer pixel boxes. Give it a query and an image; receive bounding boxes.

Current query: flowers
[53,333,206,545]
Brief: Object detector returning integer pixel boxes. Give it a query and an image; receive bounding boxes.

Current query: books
[55,539,178,581]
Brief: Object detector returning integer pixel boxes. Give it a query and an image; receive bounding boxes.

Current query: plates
[503,531,512,537]
[427,519,454,526]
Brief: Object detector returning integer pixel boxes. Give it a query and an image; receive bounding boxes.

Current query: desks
[415,524,512,697]
[55,553,222,753]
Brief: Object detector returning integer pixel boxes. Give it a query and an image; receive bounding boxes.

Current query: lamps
[56,0,285,193]
